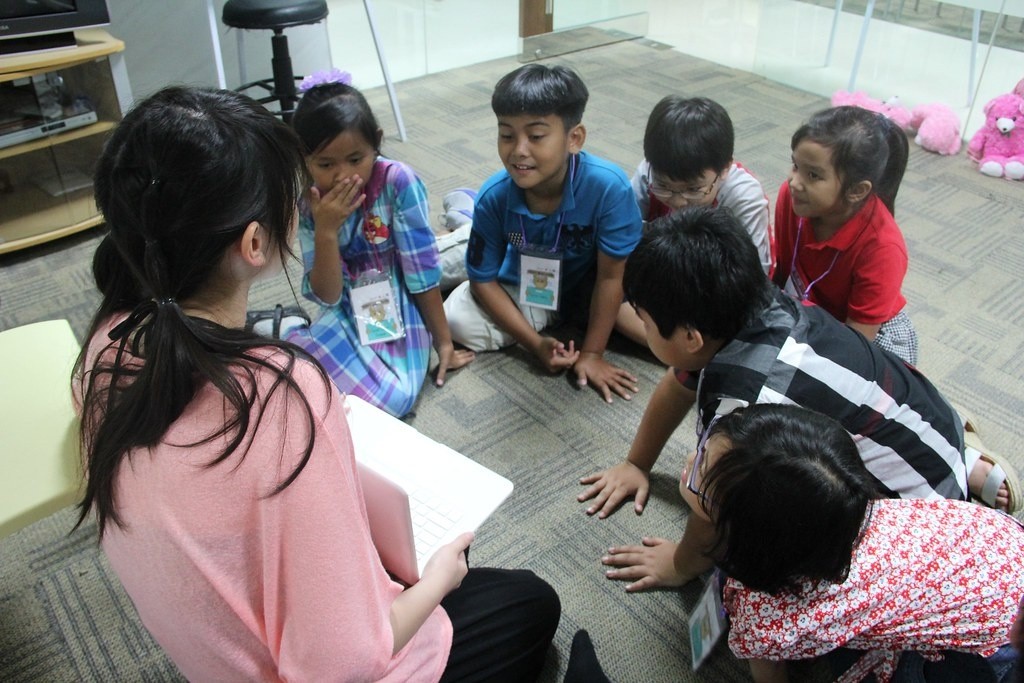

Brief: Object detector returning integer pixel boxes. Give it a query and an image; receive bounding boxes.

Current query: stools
[222,0,329,124]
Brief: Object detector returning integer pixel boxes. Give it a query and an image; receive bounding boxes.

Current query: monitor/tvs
[0,0,112,55]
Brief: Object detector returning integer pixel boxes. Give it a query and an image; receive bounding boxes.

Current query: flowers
[299,67,352,93]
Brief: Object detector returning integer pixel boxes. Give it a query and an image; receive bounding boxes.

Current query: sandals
[955,409,1023,515]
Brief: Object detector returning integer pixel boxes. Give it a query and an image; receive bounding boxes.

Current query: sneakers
[436,187,479,234]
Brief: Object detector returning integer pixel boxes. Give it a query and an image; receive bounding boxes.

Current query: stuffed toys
[911,102,962,155]
[832,89,911,133]
[966,78,1023,181]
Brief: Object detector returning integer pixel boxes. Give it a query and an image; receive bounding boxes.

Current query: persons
[67,85,562,683]
[611,95,774,352]
[575,206,968,592]
[242,70,474,419]
[678,403,1024,682]
[775,106,1023,519]
[435,65,640,408]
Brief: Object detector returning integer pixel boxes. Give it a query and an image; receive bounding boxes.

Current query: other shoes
[245,304,311,341]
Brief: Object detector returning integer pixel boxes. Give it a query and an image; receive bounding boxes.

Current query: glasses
[683,414,723,503]
[694,368,706,436]
[646,162,719,200]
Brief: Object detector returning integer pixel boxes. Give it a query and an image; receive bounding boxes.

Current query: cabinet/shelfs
[0,27,136,253]
